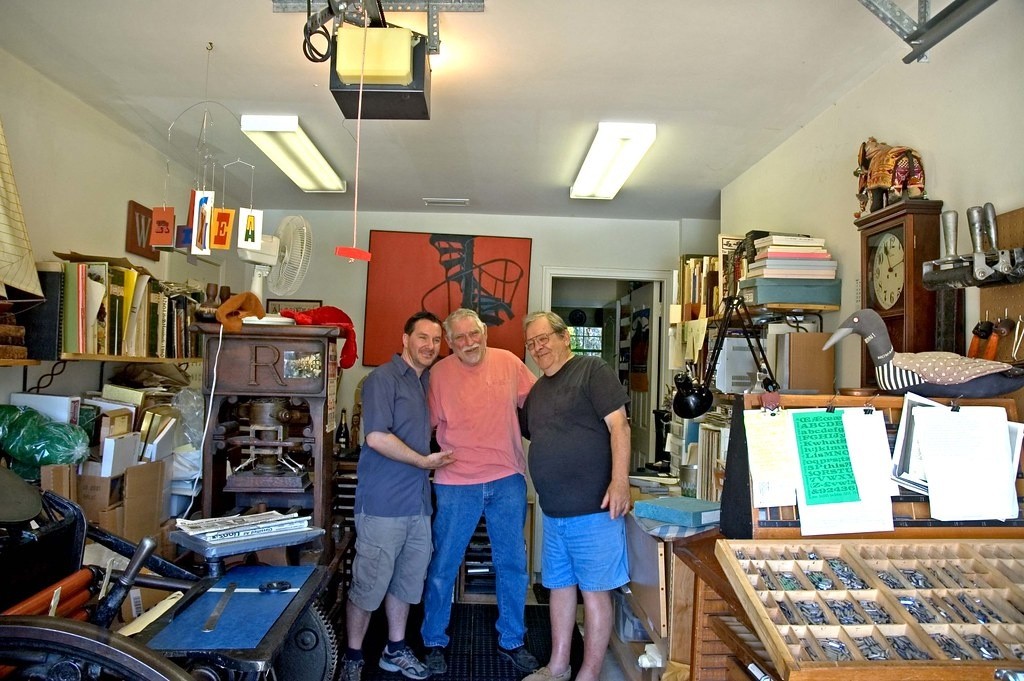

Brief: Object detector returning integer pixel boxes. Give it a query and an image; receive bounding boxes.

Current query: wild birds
[822,308,1024,398]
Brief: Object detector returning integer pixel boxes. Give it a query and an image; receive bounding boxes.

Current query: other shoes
[521,664,572,681]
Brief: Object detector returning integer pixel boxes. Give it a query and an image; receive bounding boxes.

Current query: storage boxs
[611,589,651,644]
[713,538,1024,681]
[726,656,755,681]
[738,279,842,307]
[10,383,195,621]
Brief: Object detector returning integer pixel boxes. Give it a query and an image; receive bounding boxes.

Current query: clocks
[853,198,944,387]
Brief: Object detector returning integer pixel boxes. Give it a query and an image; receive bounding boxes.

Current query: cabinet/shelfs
[332,461,539,605]
[609,254,1024,681]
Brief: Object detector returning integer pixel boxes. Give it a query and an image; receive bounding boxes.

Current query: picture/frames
[362,230,532,367]
[125,200,160,261]
[266,298,323,313]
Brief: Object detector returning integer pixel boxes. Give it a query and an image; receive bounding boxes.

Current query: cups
[676,464,698,498]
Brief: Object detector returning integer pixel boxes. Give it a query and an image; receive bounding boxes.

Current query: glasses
[525,328,559,350]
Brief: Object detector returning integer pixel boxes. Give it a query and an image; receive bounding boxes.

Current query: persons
[339,311,457,681]
[420,308,540,673]
[518,313,631,681]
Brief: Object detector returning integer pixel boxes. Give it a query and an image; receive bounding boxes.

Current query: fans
[236,215,312,325]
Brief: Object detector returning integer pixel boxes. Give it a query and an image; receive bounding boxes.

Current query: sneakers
[338,654,365,681]
[498,641,539,672]
[425,645,447,674]
[378,644,432,680]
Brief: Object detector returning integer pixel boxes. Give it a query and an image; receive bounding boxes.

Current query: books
[676,235,837,502]
[5,259,197,359]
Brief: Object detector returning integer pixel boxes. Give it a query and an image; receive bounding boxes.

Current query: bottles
[335,408,350,450]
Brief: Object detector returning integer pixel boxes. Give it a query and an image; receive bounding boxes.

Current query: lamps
[569,121,656,201]
[569,309,586,326]
[240,114,346,194]
[673,295,782,419]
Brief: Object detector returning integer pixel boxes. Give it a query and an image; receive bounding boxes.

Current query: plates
[837,387,878,397]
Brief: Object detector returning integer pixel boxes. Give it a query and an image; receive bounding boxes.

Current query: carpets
[533,583,584,604]
[362,605,584,681]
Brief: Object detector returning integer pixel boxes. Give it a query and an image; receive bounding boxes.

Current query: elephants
[853,137,926,213]
